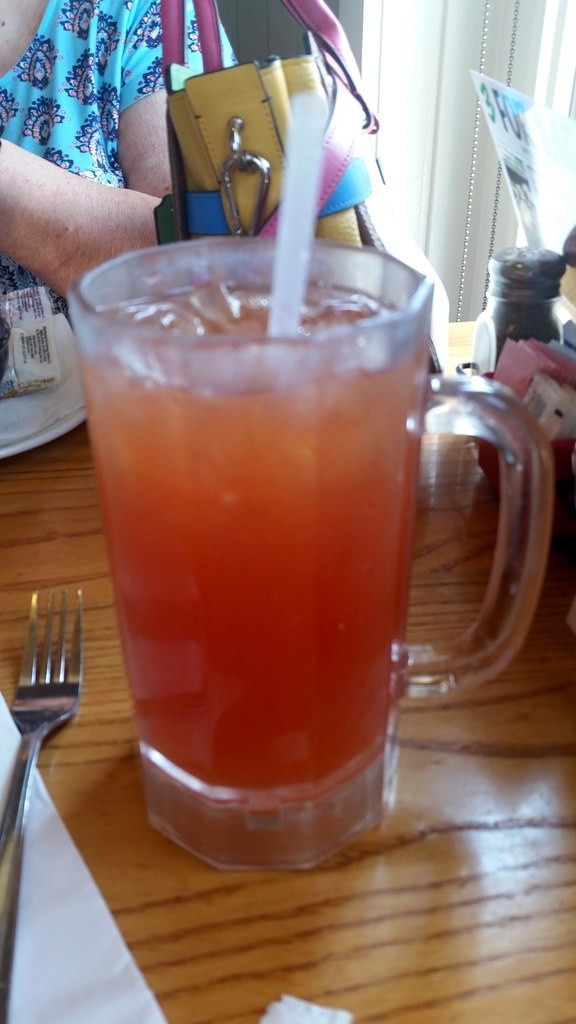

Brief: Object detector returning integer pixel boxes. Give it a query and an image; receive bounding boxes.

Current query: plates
[0,329,95,460]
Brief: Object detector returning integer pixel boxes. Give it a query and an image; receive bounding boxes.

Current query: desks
[0,318,576,1024]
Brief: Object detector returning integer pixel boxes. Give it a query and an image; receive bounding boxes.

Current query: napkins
[0,695,170,1024]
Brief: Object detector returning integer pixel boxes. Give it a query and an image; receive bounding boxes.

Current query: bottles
[470,249,568,376]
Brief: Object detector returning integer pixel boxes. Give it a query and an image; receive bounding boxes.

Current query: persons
[0,1,239,348]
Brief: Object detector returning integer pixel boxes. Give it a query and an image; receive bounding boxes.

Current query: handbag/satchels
[152,0,451,390]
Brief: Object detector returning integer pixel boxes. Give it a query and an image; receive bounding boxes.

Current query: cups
[71,230,555,873]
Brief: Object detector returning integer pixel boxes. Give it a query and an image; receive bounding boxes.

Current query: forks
[0,583,88,1024]
